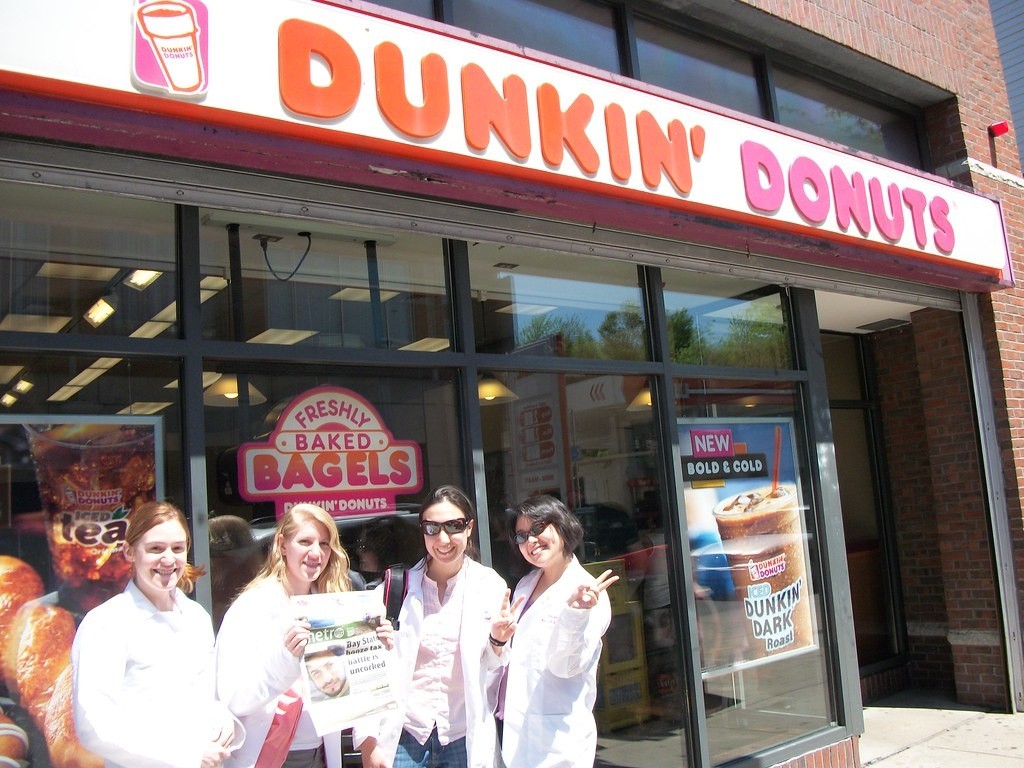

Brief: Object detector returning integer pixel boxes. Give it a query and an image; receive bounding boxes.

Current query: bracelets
[489,633,507,646]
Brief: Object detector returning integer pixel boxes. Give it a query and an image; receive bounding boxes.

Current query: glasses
[514,519,553,544]
[419,517,473,536]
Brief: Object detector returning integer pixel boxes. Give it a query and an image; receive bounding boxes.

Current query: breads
[0,555,106,768]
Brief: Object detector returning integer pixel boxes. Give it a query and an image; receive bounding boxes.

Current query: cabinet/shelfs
[583,558,652,732]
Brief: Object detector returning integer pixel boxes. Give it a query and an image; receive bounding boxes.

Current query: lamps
[477,295,518,407]
[627,377,653,411]
[204,277,266,407]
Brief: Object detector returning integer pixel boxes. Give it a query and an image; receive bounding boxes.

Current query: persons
[209,515,266,630]
[71,486,526,768]
[305,649,346,696]
[629,491,680,704]
[496,495,619,768]
[358,516,424,576]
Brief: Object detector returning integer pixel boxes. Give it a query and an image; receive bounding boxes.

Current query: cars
[207,498,677,641]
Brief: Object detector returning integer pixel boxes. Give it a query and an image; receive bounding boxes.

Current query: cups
[712,483,816,661]
[22,425,156,628]
